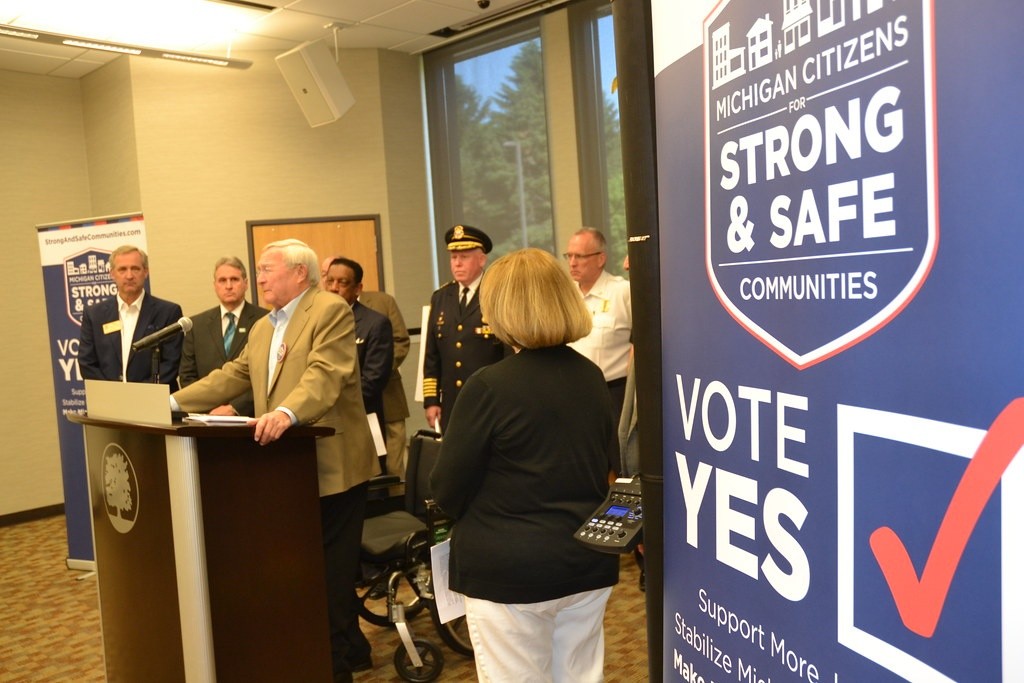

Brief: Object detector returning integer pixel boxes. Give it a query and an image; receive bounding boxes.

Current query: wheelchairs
[338,428,477,683]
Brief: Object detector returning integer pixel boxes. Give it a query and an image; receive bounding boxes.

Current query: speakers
[275,39,355,128]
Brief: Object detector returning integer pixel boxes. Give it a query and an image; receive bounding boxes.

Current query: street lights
[501,139,529,250]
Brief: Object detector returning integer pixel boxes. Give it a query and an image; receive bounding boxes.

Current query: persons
[428,248,619,683]
[320,256,410,600]
[562,230,644,591]
[179,257,273,416]
[422,225,515,441]
[170,238,382,683]
[77,247,184,394]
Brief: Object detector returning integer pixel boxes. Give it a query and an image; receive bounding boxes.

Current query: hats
[445,225,493,254]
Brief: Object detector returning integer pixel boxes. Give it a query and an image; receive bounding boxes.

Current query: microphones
[132,317,192,352]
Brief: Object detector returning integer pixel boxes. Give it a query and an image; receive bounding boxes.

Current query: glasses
[255,264,291,279]
[563,252,602,260]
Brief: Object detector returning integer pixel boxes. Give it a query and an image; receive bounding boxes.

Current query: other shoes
[346,653,373,672]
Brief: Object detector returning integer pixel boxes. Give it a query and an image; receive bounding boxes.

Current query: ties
[223,312,237,356]
[460,287,470,315]
